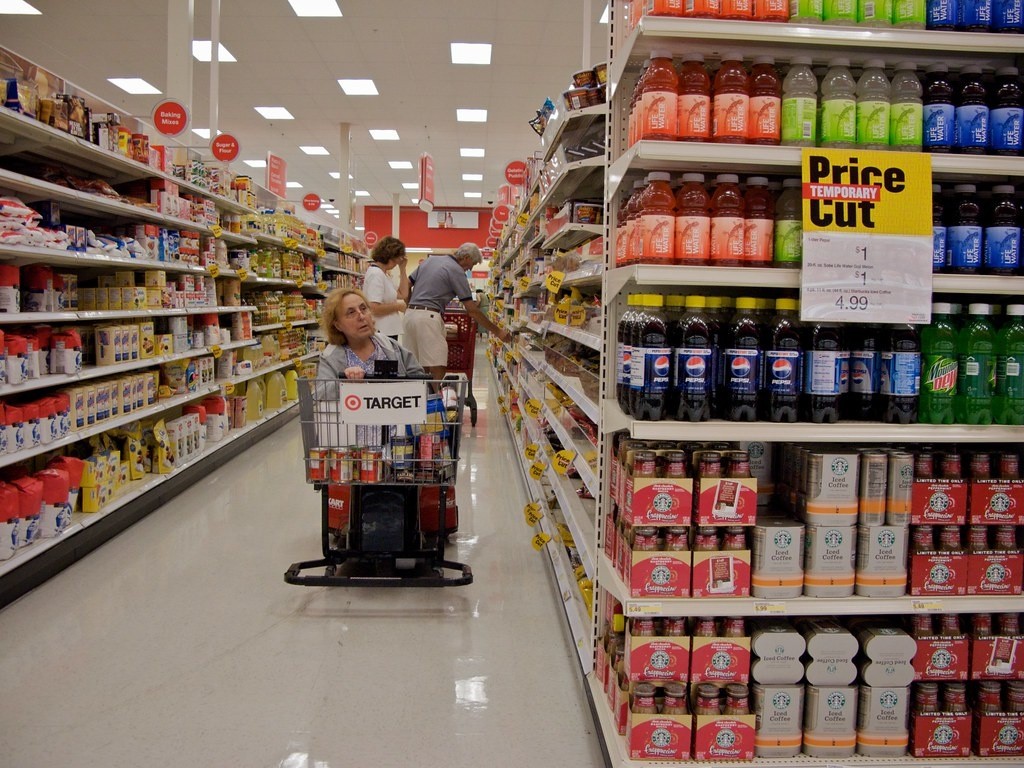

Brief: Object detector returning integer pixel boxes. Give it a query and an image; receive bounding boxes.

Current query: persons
[401,242,513,393]
[362,236,409,341]
[313,288,435,577]
[475,288,490,340]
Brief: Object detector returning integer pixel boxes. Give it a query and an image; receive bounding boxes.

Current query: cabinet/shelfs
[488,102,607,676]
[322,239,369,298]
[242,231,324,446]
[0,104,258,610]
[587,0,1024,768]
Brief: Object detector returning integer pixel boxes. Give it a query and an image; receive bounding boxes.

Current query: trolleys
[292,357,478,588]
[441,305,479,428]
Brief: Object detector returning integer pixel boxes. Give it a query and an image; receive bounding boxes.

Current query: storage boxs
[405,394,458,482]
[597,440,1024,760]
[0,80,366,513]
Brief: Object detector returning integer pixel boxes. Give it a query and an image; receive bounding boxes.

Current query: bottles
[222,214,240,235]
[627,49,1024,165]
[246,370,298,420]
[616,172,1024,275]
[237,327,306,369]
[248,209,307,244]
[243,290,313,327]
[437,211,453,228]
[249,246,322,283]
[615,293,1024,425]
[630,0,1024,33]
[314,298,325,318]
[216,237,228,269]
[117,130,152,165]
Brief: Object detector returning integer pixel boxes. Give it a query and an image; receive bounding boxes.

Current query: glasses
[337,303,372,319]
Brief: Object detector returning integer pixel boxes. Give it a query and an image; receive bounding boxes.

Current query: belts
[407,304,440,313]
